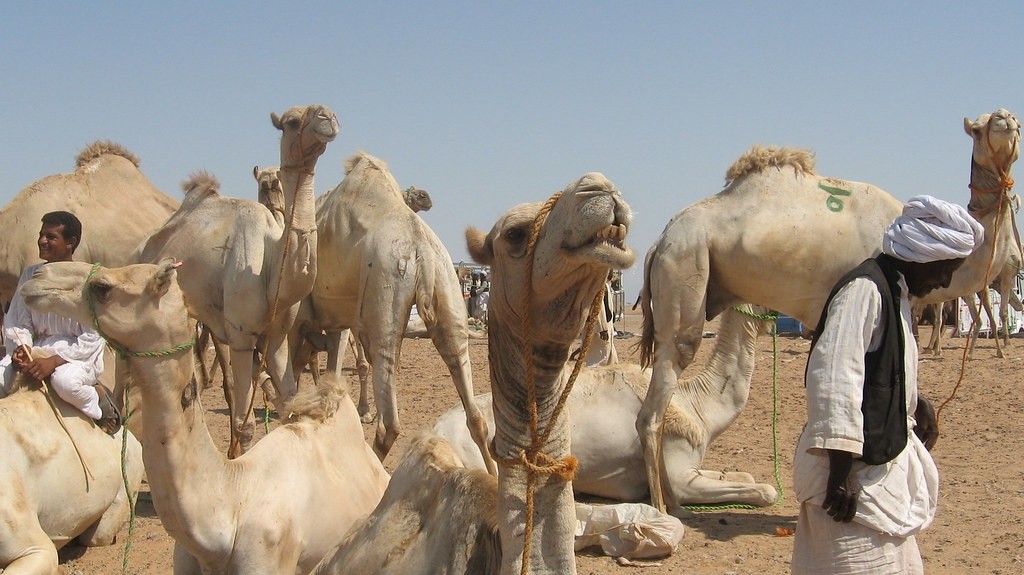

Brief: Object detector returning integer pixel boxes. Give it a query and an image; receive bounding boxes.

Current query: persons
[0,211,123,436]
[582,269,618,368]
[631,287,644,311]
[475,271,489,321]
[791,194,985,575]
[467,271,482,318]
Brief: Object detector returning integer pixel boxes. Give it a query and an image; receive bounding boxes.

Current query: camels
[632,107,1023,521]
[0,104,778,574]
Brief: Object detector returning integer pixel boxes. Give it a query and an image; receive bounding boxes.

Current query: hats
[882,194,988,263]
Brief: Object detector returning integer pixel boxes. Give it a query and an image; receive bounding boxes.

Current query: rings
[36,371,40,375]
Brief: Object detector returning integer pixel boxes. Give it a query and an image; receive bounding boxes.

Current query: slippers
[93,382,122,435]
